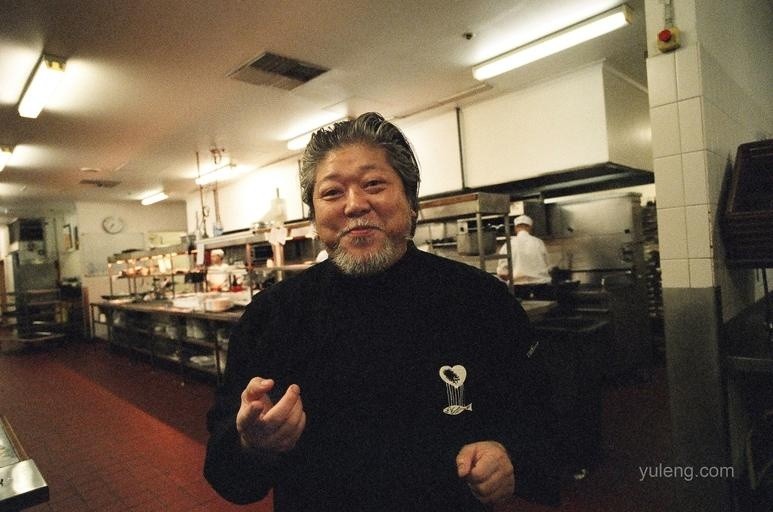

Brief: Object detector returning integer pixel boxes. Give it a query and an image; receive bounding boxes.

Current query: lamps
[139,192,169,208]
[284,116,354,151]
[194,163,245,190]
[0,51,66,172]
[470,5,634,83]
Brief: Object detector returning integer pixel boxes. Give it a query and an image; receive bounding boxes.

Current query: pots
[454,230,496,256]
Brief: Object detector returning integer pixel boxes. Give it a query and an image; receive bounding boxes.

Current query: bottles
[271,188,286,221]
[213,216,224,236]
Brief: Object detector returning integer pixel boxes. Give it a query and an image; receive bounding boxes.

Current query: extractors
[181,61,659,241]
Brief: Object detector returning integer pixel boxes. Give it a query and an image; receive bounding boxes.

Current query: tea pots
[54,276,80,298]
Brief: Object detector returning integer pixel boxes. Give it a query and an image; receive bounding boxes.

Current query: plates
[152,312,208,339]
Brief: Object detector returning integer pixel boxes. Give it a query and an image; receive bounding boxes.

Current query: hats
[211,249,225,257]
[514,216,534,227]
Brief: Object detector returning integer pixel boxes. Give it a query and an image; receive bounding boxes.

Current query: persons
[266,253,274,266]
[205,248,236,287]
[200,111,563,510]
[497,214,552,299]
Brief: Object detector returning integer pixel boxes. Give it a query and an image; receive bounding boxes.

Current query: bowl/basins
[180,235,195,243]
[204,298,228,313]
